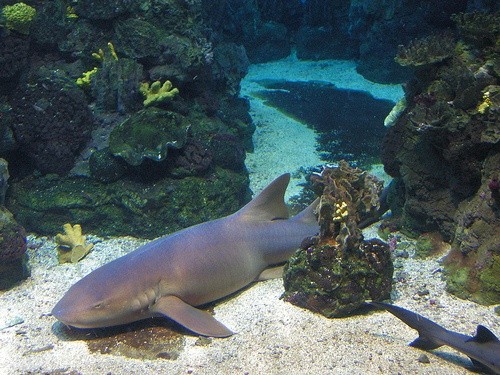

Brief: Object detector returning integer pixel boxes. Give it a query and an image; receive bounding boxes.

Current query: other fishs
[363,299,500,375]
[49,172,395,340]
